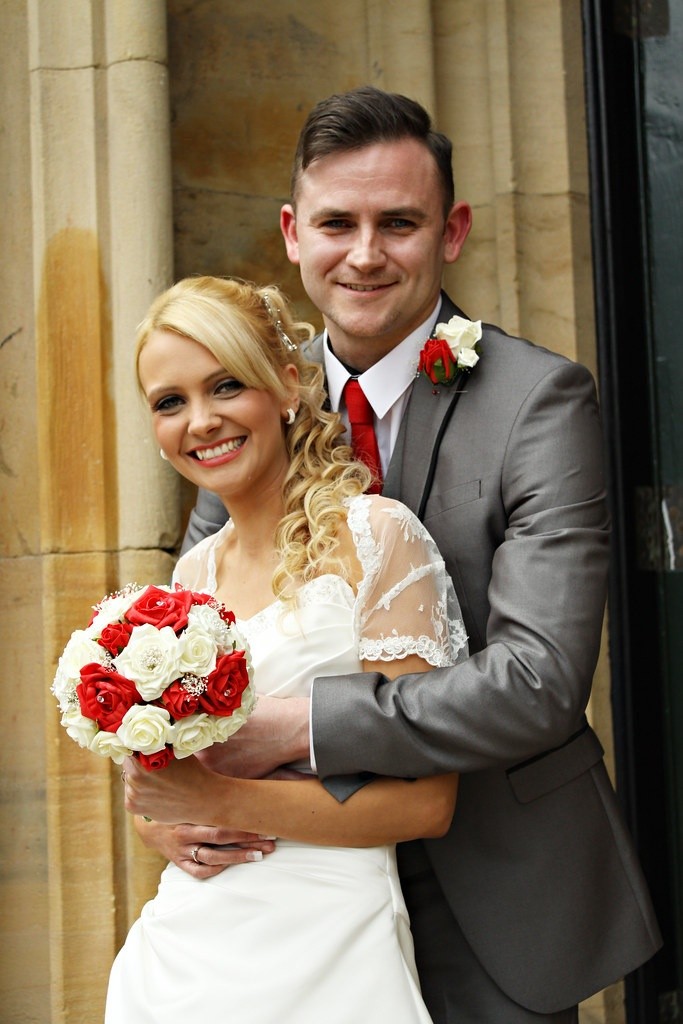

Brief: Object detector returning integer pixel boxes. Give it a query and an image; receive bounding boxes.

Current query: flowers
[50,582,256,823]
[413,315,482,395]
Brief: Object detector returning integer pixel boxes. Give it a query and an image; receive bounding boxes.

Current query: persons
[179,89,663,1024]
[105,275,471,1024]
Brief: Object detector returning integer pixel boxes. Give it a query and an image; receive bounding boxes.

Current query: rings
[121,769,126,783]
[191,847,201,863]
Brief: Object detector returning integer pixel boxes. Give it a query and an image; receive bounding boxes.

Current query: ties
[344,379,383,496]
